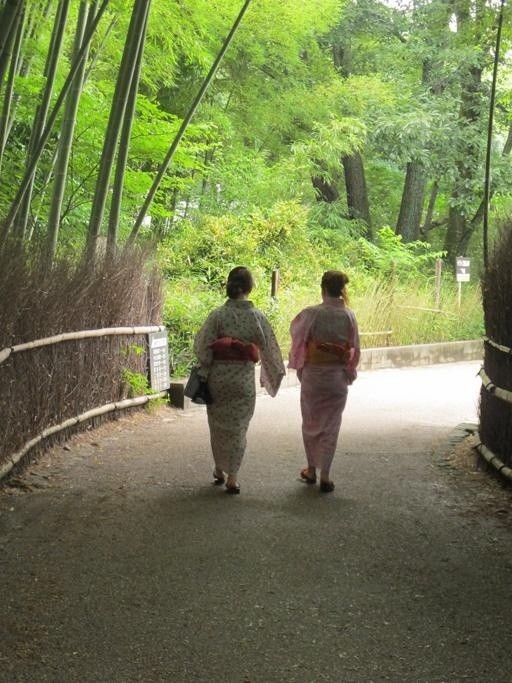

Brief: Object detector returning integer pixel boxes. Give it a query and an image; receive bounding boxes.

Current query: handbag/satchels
[184,366,213,404]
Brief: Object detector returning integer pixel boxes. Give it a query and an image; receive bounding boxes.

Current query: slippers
[320,481,335,491]
[301,468,316,484]
[213,470,225,485]
[226,482,240,492]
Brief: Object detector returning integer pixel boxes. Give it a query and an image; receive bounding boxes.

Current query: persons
[286,268,362,492]
[187,265,288,492]
